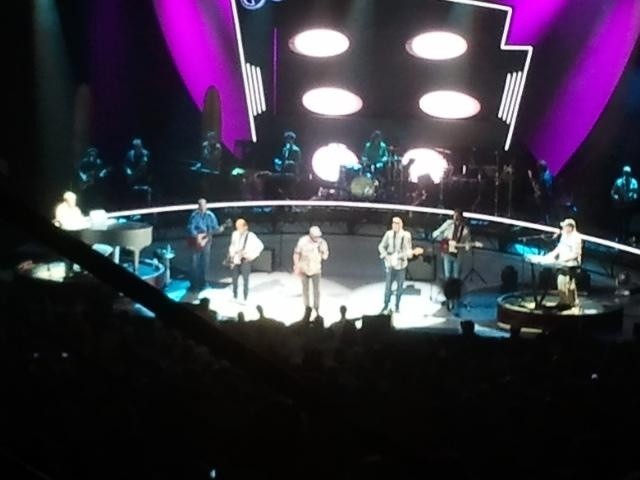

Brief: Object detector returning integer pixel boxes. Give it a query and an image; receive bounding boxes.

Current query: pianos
[525,255,582,276]
[62,210,152,277]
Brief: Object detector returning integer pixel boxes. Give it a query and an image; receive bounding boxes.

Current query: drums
[351,175,376,200]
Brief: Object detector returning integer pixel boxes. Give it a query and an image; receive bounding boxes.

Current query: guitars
[440,238,483,254]
[380,247,424,267]
[221,249,251,268]
[188,218,233,251]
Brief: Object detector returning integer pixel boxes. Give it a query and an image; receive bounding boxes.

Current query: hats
[560,218,576,227]
[309,226,322,237]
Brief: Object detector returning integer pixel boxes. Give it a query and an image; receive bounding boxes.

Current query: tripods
[265,229,292,274]
[461,230,488,285]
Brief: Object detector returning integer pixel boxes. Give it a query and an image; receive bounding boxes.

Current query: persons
[0,131,640,479]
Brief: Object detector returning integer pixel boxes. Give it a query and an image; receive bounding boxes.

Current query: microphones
[552,230,561,240]
